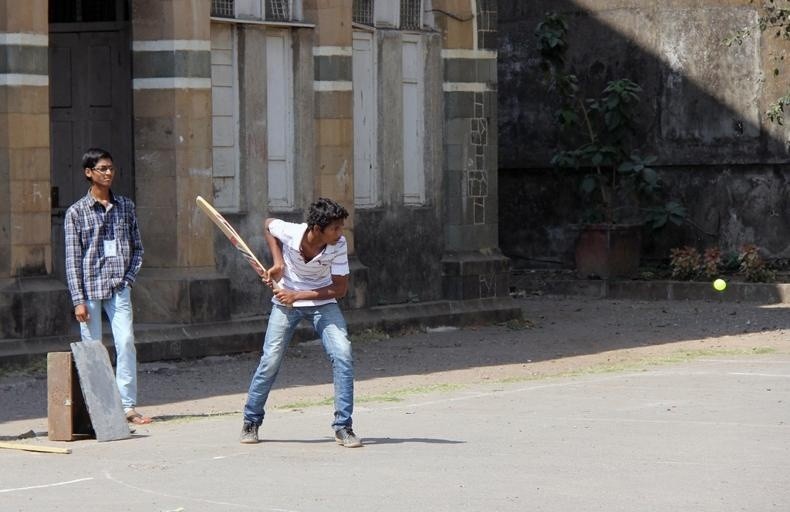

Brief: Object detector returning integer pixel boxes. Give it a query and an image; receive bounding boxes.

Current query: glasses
[91,166,114,171]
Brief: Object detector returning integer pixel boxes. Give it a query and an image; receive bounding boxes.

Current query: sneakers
[336,428,362,447]
[241,422,259,443]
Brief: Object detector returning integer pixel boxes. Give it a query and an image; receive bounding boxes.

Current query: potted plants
[535,10,688,281]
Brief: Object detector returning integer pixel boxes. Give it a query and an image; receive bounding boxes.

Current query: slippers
[126,414,152,424]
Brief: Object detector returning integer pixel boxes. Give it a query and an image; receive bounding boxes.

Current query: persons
[63,147,152,424]
[241,197,363,447]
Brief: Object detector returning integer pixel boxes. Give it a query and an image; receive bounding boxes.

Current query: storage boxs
[47,352,93,442]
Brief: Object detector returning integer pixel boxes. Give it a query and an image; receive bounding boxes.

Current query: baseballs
[714,278,727,292]
